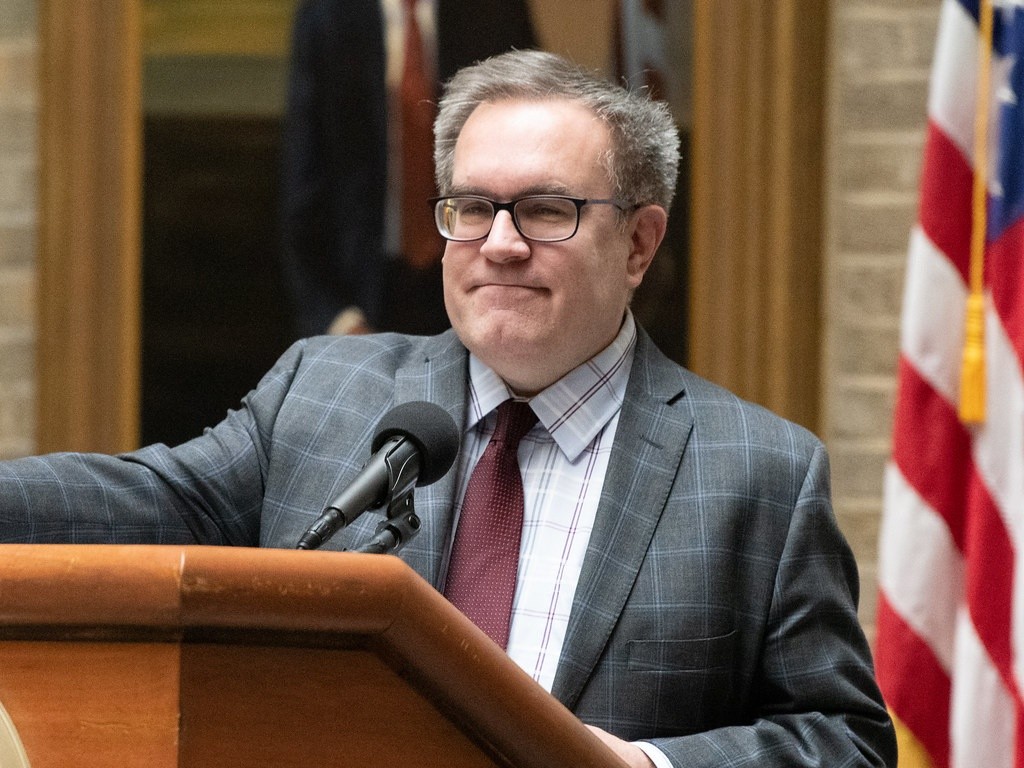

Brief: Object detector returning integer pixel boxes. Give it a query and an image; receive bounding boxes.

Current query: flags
[875,0,1024,768]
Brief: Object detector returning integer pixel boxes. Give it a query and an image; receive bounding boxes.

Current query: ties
[444,402,540,656]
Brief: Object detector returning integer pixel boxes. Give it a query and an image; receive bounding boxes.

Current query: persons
[2,47,902,768]
[273,0,557,377]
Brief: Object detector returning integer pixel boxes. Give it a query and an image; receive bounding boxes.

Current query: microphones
[294,402,459,551]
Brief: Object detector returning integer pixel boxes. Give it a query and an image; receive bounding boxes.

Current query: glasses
[428,195,635,243]
